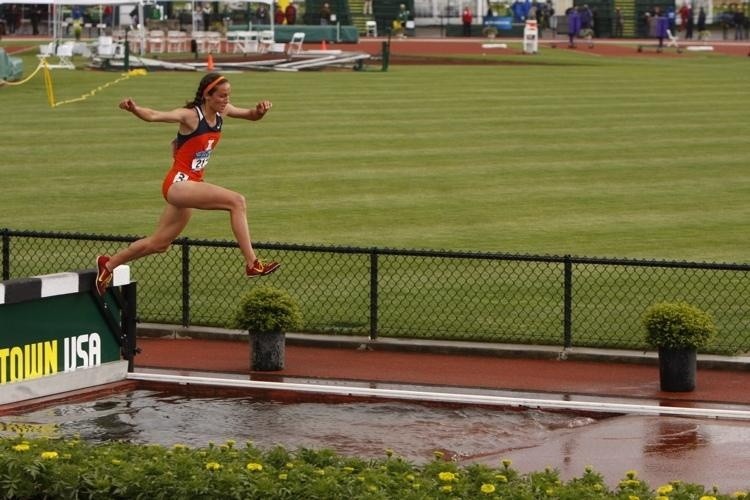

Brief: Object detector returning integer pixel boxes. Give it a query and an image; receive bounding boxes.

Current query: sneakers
[246,259,280,278]
[95,255,113,295]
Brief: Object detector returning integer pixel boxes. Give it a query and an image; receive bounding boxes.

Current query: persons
[1,4,47,37]
[93,72,281,297]
[191,1,411,38]
[503,0,749,50]
[457,5,473,37]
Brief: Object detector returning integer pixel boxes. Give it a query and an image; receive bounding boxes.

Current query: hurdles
[0,264,141,409]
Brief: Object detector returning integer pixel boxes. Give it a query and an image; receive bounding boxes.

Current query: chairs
[36,23,310,74]
[364,18,381,40]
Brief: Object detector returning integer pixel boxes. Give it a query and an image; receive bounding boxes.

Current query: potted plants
[642,302,715,393]
[234,290,301,371]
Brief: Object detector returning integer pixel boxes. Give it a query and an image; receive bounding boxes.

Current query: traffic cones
[321,38,330,50]
[205,52,216,70]
